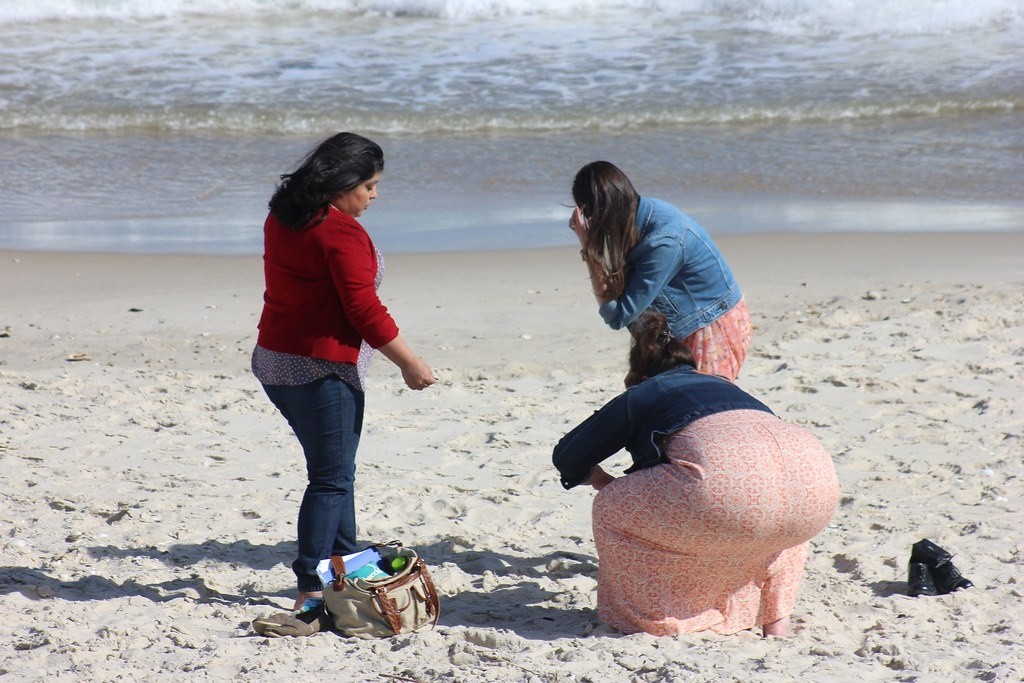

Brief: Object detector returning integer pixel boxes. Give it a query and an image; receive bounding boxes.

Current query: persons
[253,131,436,610]
[552,314,840,637]
[569,161,752,384]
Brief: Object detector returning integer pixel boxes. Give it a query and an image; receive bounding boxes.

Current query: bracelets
[580,249,588,260]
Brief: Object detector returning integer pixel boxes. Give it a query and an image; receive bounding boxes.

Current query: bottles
[392,557,408,576]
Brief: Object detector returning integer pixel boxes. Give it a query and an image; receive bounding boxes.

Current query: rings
[571,224,576,228]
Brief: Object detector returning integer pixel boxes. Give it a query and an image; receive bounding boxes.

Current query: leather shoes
[932,554,975,594]
[907,555,937,595]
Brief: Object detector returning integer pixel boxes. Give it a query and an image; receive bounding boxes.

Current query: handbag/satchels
[321,539,441,641]
